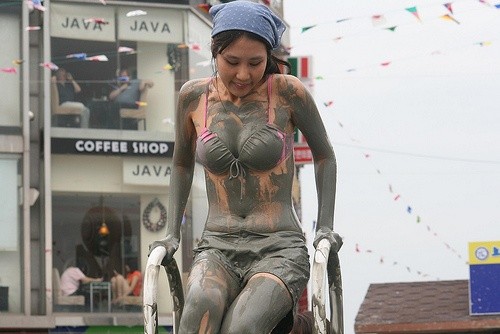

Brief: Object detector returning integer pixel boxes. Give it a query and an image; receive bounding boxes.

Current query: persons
[111,259,142,305]
[56,68,90,128]
[148,0,343,334]
[60,258,103,312]
[109,69,154,130]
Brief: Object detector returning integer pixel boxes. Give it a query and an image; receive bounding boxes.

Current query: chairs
[112,272,143,313]
[120,79,154,130]
[52,267,85,312]
[50,76,81,127]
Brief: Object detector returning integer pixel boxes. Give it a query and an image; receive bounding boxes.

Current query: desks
[90,281,111,312]
